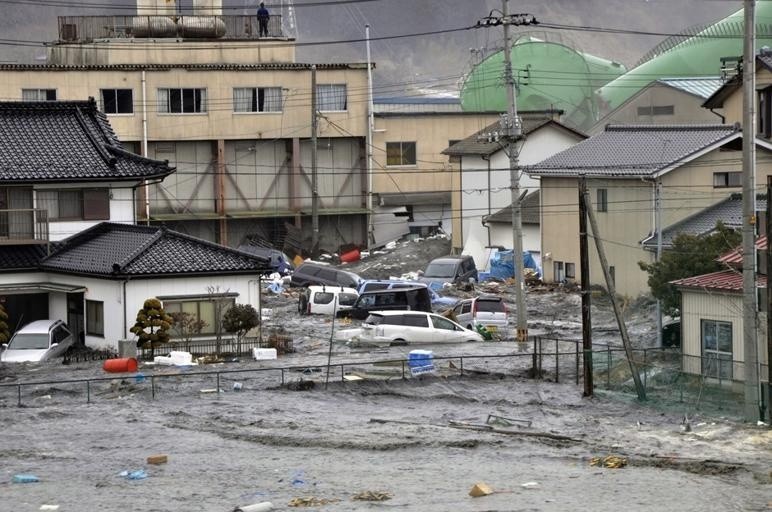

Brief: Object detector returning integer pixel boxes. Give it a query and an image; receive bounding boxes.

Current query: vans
[239,244,508,346]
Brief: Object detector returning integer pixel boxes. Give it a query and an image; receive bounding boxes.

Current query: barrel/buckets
[340,249,360,263]
[103,357,138,373]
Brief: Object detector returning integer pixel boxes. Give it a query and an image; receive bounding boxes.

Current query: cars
[0,320,76,363]
